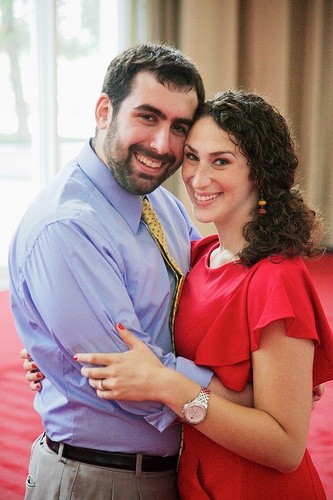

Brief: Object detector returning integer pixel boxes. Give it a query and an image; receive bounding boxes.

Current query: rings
[98,378,106,392]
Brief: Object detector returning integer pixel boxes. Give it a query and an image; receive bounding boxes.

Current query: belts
[46,436,178,472]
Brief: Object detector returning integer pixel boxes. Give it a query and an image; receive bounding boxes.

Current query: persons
[20,88,333,500]
[7,43,325,500]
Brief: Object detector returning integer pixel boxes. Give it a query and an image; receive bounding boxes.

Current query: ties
[141,198,185,476]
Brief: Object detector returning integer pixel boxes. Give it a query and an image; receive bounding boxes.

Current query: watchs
[179,386,211,425]
